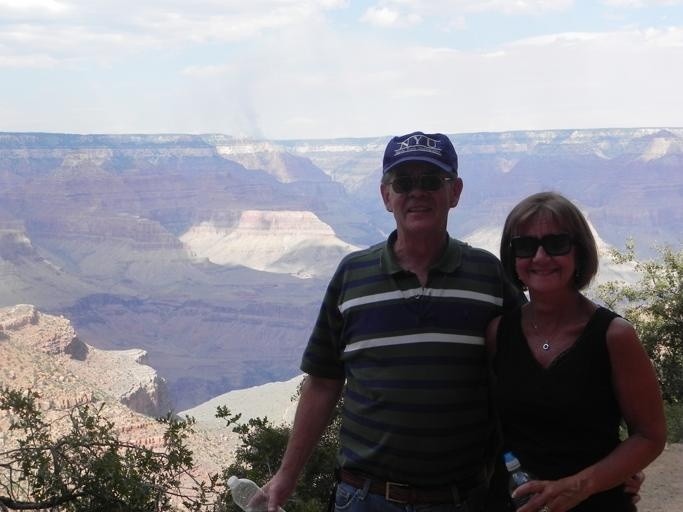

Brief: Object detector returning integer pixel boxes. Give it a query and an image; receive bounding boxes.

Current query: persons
[487,191,665,511]
[248,132,643,511]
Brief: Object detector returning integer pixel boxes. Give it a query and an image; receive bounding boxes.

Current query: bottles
[225,475,285,511]
[502,451,547,512]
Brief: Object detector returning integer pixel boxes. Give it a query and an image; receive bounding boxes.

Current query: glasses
[385,176,454,194]
[508,231,574,259]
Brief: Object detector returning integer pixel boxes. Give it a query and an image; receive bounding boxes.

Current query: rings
[542,504,549,511]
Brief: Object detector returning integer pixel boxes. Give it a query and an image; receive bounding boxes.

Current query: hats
[383,132,458,174]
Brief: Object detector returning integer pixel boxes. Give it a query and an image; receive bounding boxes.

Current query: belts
[337,465,468,505]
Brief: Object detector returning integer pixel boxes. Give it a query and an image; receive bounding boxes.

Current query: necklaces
[523,304,588,349]
